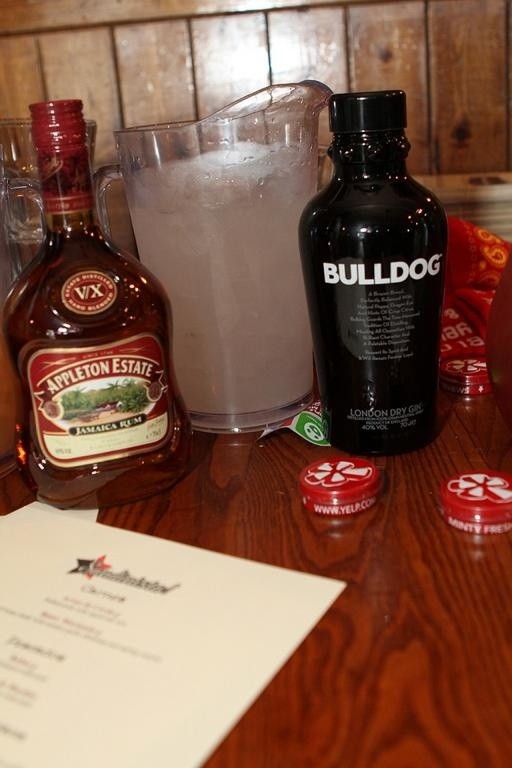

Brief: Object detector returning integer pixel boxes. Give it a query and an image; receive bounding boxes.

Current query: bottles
[293,91,448,454]
[2,98,195,510]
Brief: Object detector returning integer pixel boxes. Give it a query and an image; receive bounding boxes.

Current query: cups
[1,114,103,277]
